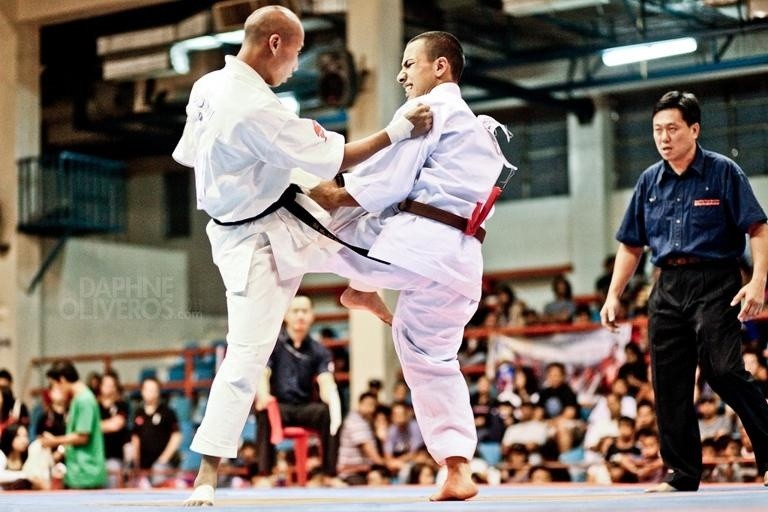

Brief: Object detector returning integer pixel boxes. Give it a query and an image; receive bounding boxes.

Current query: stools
[271,426,323,487]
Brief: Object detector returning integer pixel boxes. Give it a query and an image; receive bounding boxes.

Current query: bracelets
[384,114,415,146]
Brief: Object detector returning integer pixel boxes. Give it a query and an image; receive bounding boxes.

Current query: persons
[599,89,766,492]
[172,4,433,505]
[309,31,519,499]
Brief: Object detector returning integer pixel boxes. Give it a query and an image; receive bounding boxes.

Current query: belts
[665,258,700,264]
